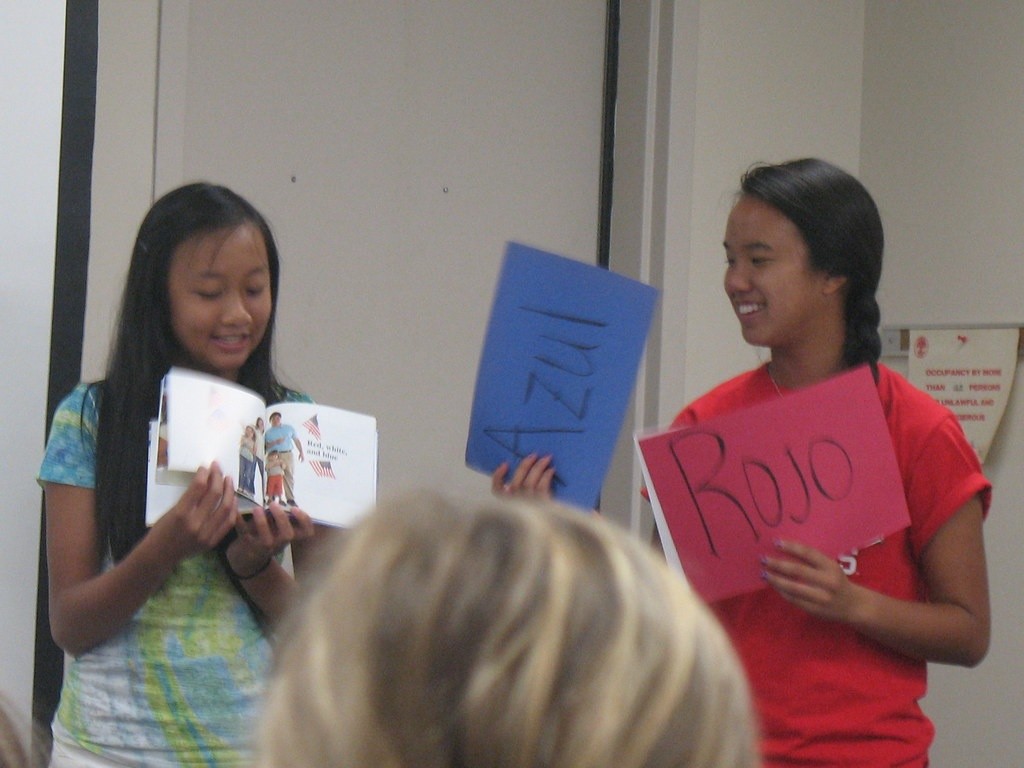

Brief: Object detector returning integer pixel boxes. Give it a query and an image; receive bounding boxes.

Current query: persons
[235,412,305,509]
[257,483,762,768]
[36,183,330,768]
[491,158,995,768]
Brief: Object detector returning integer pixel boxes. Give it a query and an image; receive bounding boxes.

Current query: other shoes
[287,500,298,507]
[279,501,286,506]
[267,497,275,506]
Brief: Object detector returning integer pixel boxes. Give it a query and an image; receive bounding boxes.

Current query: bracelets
[237,556,272,581]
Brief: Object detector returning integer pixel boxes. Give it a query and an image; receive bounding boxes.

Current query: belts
[272,450,292,453]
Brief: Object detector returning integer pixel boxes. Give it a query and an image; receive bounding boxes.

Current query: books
[144,366,378,534]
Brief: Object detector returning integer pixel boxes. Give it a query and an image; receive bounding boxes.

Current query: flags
[303,414,320,440]
[309,460,337,480]
